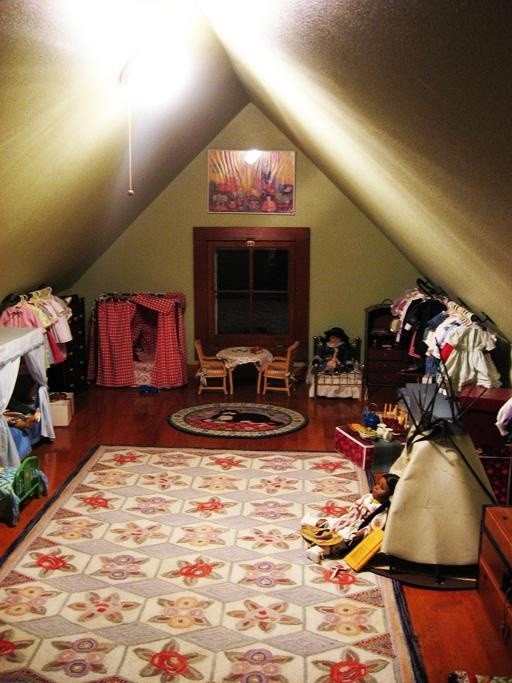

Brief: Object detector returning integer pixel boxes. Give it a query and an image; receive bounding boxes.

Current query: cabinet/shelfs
[364,304,427,408]
[48,295,88,399]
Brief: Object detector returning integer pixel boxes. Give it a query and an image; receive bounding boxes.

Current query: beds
[1,327,51,464]
[307,335,366,402]
[94,294,187,389]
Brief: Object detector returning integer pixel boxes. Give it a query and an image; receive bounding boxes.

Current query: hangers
[10,287,55,308]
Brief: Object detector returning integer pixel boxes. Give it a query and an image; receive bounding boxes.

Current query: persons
[304,474,399,570]
[312,327,355,372]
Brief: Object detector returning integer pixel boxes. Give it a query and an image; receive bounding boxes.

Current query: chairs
[1,455,43,524]
[193,339,300,396]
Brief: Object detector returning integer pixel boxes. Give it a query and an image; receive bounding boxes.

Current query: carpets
[1,444,427,683]
[167,401,309,439]
